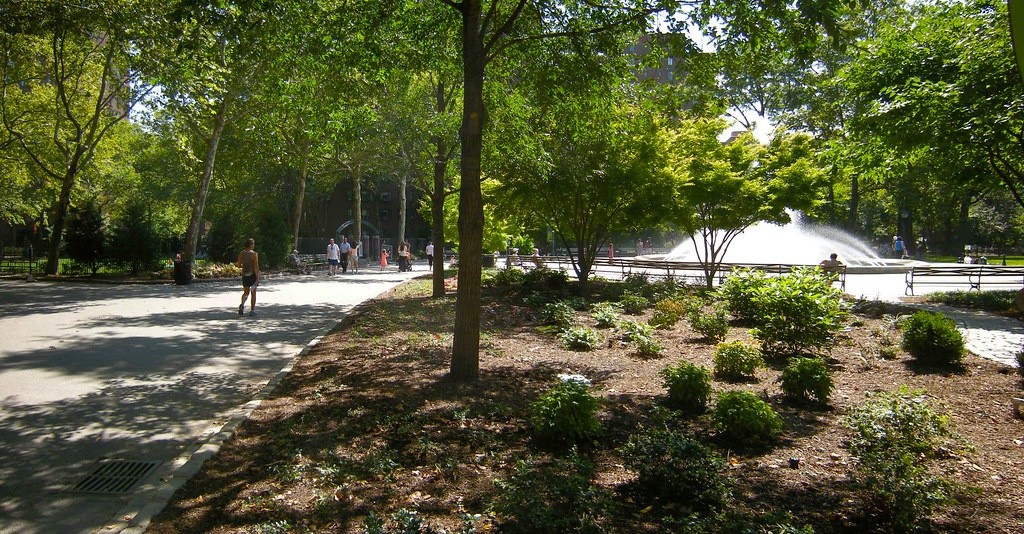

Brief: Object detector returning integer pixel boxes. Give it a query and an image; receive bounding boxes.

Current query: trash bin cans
[174,260,194,285]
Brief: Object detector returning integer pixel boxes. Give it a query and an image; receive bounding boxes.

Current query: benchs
[904,265,1023,296]
[505,255,846,292]
[288,253,329,272]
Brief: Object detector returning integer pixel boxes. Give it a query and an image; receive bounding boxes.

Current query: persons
[450,254,456,266]
[512,248,527,271]
[339,238,351,274]
[381,249,388,271]
[889,234,912,261]
[531,249,542,268]
[291,249,308,274]
[820,254,842,280]
[963,249,974,265]
[350,245,358,274]
[397,238,416,268]
[237,237,263,316]
[608,244,613,259]
[326,238,340,276]
[425,241,434,272]
